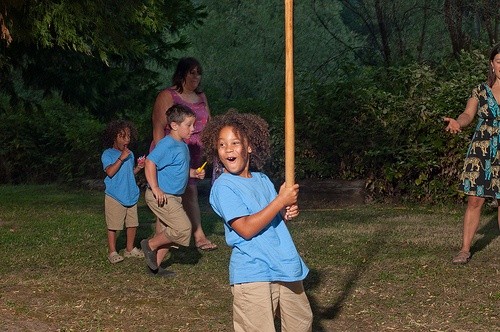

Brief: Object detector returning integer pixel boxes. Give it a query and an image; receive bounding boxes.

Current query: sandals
[123,247,145,258]
[106,251,123,264]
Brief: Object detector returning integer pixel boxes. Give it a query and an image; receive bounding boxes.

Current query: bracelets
[118,158,123,162]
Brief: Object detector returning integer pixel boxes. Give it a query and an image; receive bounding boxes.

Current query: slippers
[195,240,218,251]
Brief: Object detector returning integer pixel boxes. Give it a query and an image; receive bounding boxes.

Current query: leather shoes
[140,239,158,270]
[143,264,176,277]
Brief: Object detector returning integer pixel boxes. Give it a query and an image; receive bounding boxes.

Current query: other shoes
[453,250,471,264]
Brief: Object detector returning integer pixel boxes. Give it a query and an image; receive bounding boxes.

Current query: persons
[140,104,206,278]
[149,57,218,251]
[444,47,500,264]
[101,120,145,264]
[198,108,313,332]
